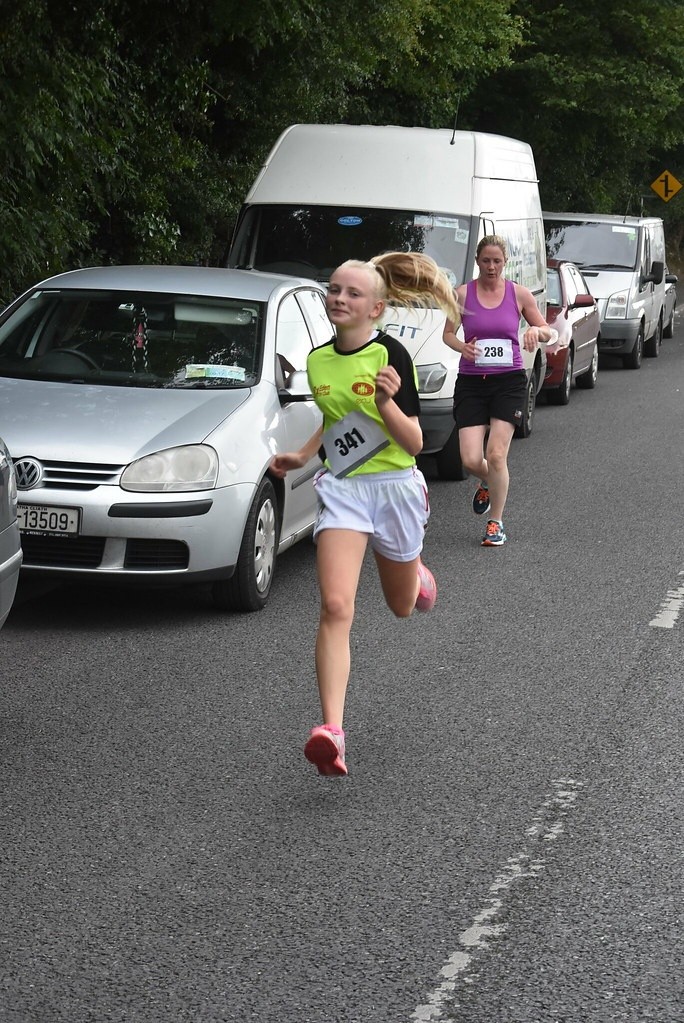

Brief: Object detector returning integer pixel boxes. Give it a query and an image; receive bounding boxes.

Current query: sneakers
[301,723,349,777]
[471,480,491,515]
[415,556,437,613]
[479,519,507,546]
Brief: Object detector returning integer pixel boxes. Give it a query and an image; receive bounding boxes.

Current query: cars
[0,260,340,609]
[665,265,680,336]
[541,258,602,403]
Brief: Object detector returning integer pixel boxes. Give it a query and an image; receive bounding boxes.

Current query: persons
[268,250,473,776]
[442,235,553,545]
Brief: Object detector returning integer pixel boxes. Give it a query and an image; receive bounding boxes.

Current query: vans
[533,213,665,368]
[219,84,551,478]
